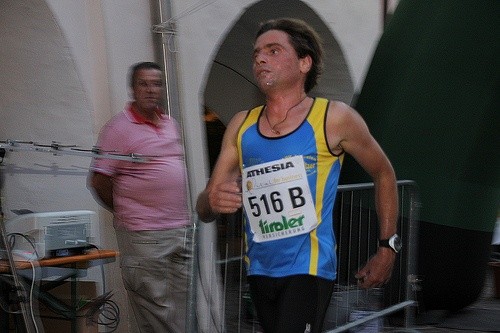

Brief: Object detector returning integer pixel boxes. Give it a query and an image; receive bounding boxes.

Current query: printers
[0,209,98,262]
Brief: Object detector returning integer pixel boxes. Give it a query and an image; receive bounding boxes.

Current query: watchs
[377,233,403,253]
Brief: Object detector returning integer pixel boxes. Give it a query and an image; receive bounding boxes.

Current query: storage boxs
[8,280,98,333]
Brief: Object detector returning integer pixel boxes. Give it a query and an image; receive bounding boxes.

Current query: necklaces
[264,93,307,134]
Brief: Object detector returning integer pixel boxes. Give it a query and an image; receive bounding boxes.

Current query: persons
[194,17,403,333]
[87,61,192,332]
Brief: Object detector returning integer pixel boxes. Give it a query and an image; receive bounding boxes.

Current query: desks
[0,250,117,333]
